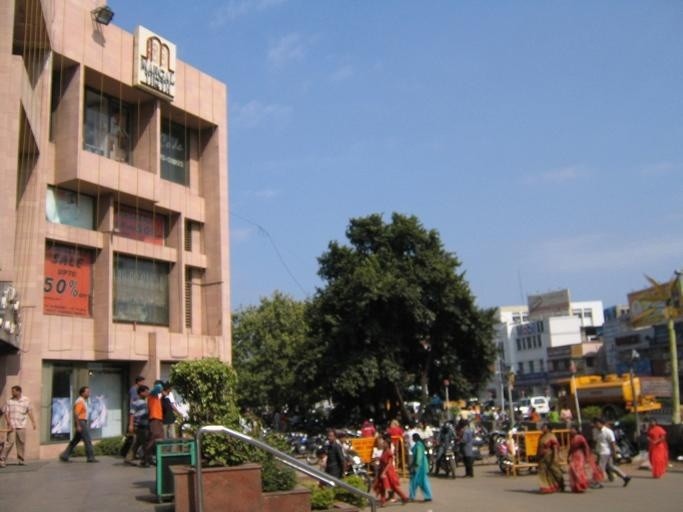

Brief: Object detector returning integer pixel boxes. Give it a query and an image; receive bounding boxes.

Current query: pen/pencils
[515,396,549,418]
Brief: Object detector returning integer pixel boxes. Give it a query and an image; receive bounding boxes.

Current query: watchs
[342,442,371,493]
[421,436,456,479]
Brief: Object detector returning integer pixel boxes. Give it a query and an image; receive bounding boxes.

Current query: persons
[0,385,38,468]
[646,417,667,479]
[483,402,631,493]
[58,385,100,465]
[117,377,188,468]
[315,403,482,507]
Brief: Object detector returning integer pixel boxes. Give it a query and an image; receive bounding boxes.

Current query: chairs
[87,459,98,462]
[623,476,630,487]
[60,457,71,463]
[123,454,153,468]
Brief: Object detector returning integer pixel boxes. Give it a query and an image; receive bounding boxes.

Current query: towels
[155,438,196,503]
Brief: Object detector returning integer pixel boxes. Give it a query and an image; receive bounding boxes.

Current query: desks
[629,350,642,451]
[444,380,451,419]
[569,360,583,432]
